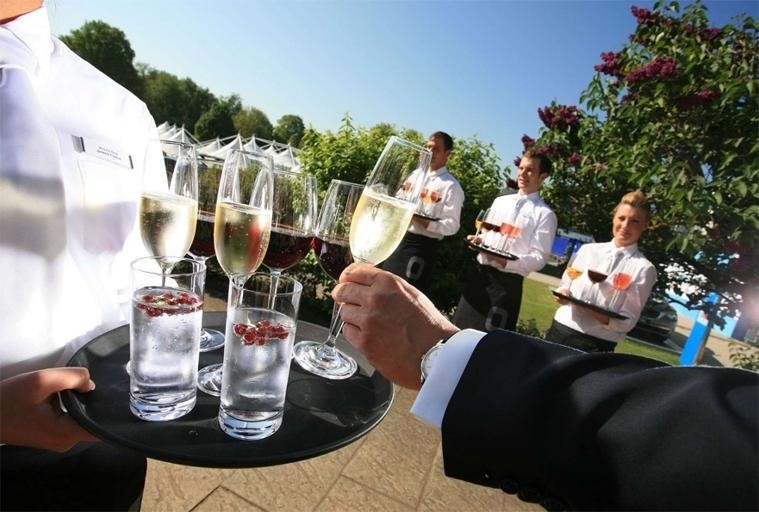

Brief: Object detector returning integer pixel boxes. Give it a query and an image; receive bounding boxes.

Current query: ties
[512,198,525,224]
[605,251,625,276]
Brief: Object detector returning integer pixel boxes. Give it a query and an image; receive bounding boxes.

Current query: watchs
[419,336,447,384]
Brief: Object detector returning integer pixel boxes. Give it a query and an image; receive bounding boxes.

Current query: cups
[130,287,197,421]
[219,273,303,442]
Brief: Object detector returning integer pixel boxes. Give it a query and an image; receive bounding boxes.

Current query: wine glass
[170,157,225,353]
[249,168,319,310]
[125,139,198,386]
[583,262,608,304]
[434,191,442,206]
[471,209,521,254]
[604,266,633,311]
[313,177,366,337]
[399,181,433,217]
[292,137,434,381]
[562,255,583,298]
[198,152,275,396]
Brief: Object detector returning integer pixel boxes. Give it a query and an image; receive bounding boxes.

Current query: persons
[451,148,559,332]
[543,188,658,353]
[0,0,183,511]
[377,130,465,307]
[330,260,759,511]
